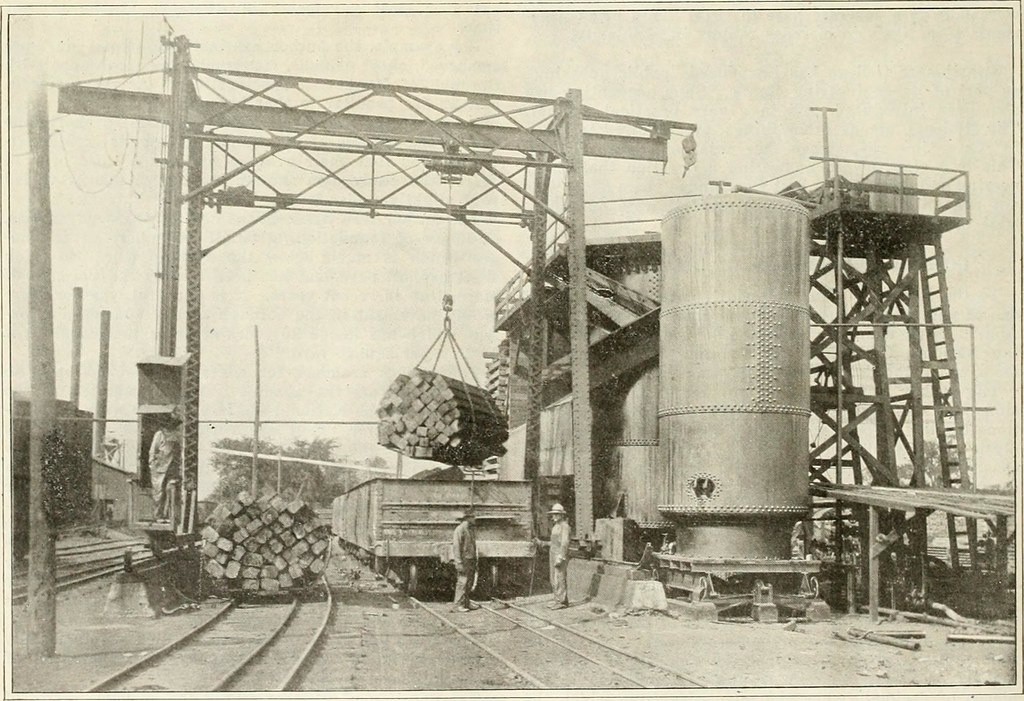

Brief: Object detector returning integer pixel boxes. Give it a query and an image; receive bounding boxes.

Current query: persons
[453,510,480,613]
[531,503,572,611]
[147,413,183,504]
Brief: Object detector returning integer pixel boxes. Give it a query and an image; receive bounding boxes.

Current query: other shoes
[464,602,478,610]
[453,606,469,612]
[547,602,568,610]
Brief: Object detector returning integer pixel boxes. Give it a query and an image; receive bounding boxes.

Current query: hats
[549,503,566,514]
